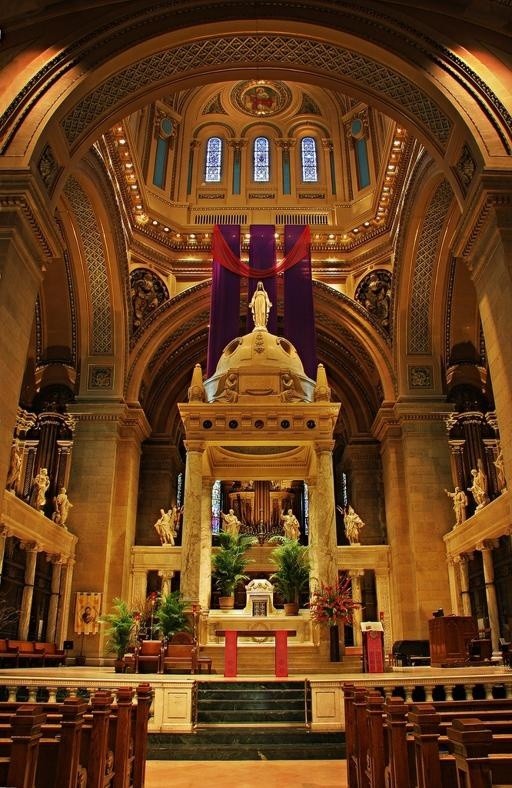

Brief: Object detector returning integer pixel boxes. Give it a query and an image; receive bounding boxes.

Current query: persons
[252,89,272,110]
[249,281,272,326]
[7,444,22,496]
[467,458,487,509]
[281,374,294,402]
[82,607,94,624]
[53,488,73,530]
[224,374,237,402]
[444,487,468,525]
[219,509,241,534]
[35,467,50,515]
[135,273,158,319]
[365,275,391,319]
[281,509,300,540]
[154,505,177,545]
[495,441,506,490]
[344,508,365,543]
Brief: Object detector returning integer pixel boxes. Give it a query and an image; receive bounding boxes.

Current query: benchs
[0,678,154,788]
[337,681,512,788]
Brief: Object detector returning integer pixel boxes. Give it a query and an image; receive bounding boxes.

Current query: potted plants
[209,528,259,610]
[95,595,140,674]
[265,535,318,616]
[142,590,198,658]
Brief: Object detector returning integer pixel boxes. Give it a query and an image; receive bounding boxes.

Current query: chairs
[135,603,200,674]
[0,639,67,668]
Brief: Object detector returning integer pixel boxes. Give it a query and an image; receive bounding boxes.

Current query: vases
[330,625,340,662]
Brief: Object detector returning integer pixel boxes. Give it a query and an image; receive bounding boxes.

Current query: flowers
[302,574,368,627]
